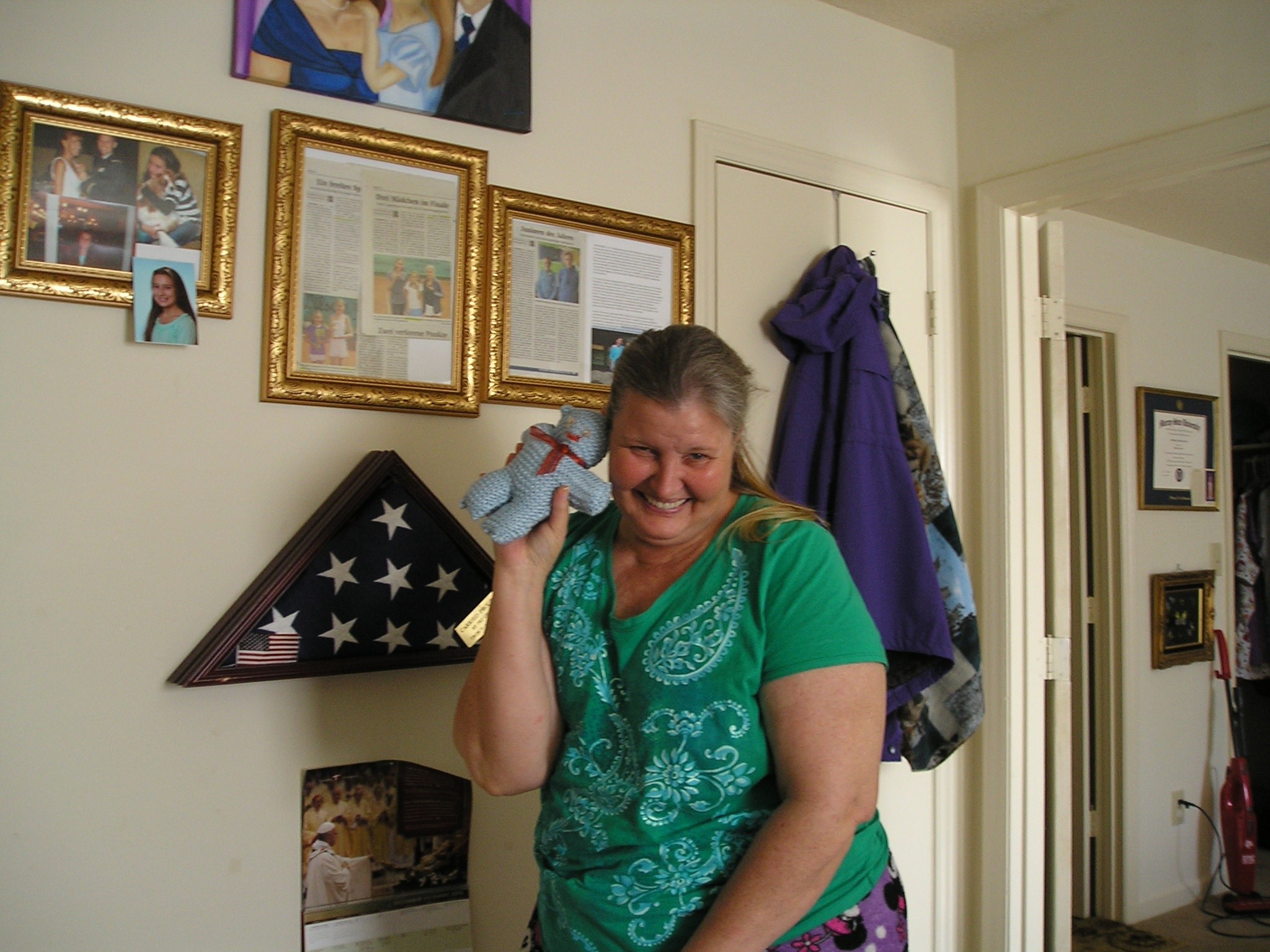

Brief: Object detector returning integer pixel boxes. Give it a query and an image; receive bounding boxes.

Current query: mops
[1214,630,1270,915]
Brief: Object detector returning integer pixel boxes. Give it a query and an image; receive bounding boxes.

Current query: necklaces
[323,0,350,10]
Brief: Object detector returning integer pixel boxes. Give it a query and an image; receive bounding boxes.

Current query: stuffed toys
[463,406,613,545]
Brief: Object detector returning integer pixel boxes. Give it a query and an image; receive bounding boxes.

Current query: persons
[302,771,461,911]
[387,259,444,318]
[48,131,202,267]
[306,310,330,363]
[143,267,198,345]
[535,257,557,299]
[1164,593,1199,645]
[454,325,908,952]
[557,250,579,303]
[248,0,531,134]
[328,298,353,365]
[608,338,625,371]
